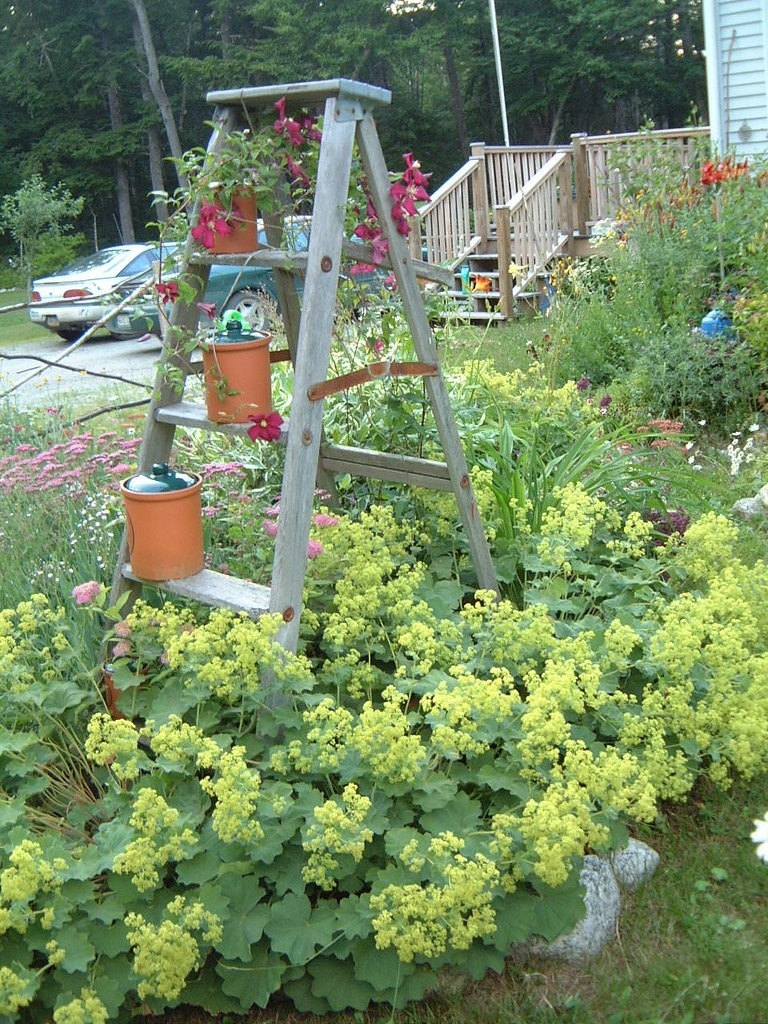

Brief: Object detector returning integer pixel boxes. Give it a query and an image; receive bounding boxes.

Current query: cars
[27,240,188,343]
[104,234,445,342]
[256,215,317,243]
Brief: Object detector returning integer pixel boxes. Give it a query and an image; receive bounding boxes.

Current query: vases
[91,179,275,723]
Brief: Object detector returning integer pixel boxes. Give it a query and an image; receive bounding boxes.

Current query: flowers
[152,92,433,448]
